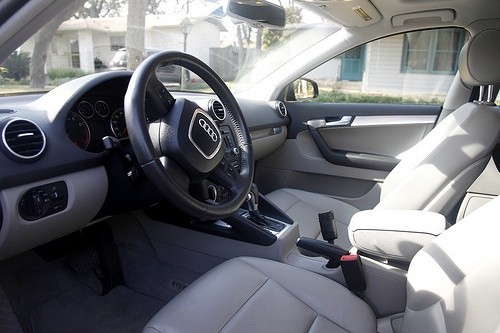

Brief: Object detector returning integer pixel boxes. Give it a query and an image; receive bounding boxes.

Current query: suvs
[109,46,189,83]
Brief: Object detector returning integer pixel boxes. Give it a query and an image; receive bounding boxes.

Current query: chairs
[264,26,499,258]
[142,192,500,333]
